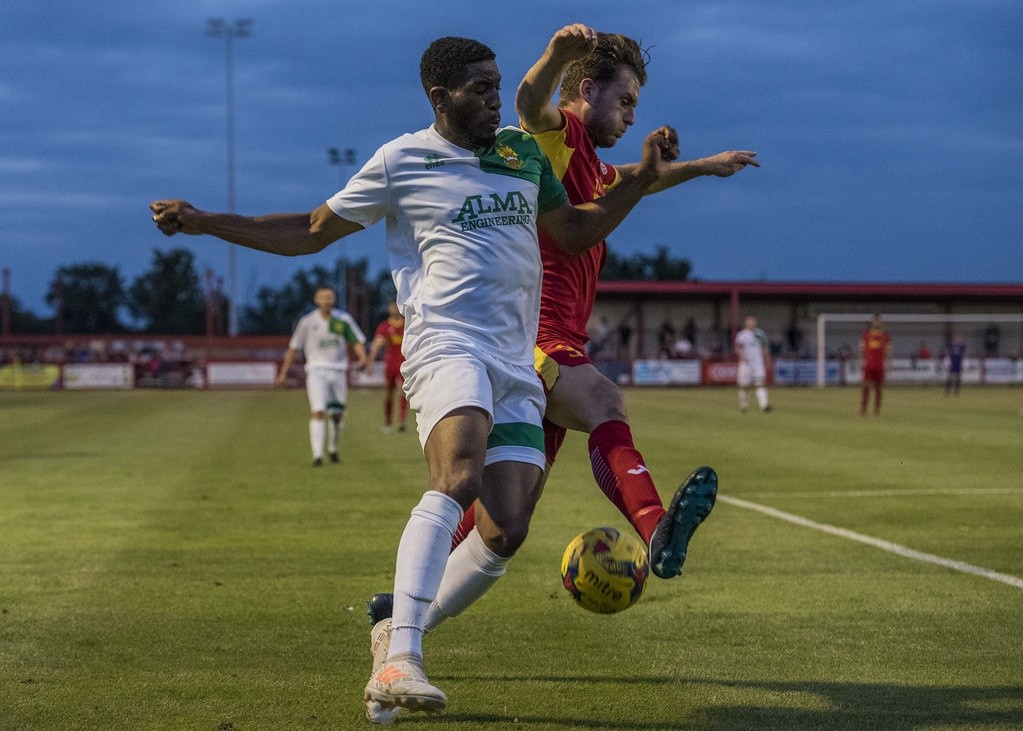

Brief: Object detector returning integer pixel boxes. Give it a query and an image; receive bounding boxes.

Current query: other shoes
[327,450,339,462]
[382,426,392,432]
[760,404,772,412]
[397,424,405,432]
[314,457,322,464]
[740,402,749,413]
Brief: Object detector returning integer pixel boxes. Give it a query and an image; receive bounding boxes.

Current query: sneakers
[368,592,393,625]
[364,651,448,713]
[365,618,403,724]
[645,466,719,579]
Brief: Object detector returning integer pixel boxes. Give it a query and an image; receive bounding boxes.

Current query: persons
[587,314,817,361]
[835,342,855,360]
[156,35,681,724]
[916,342,931,358]
[856,313,896,417]
[366,299,411,434]
[368,23,760,628]
[984,322,1002,357]
[276,285,368,468]
[734,316,776,414]
[942,333,968,399]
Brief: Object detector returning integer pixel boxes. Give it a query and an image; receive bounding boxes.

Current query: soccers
[559,524,651,617]
